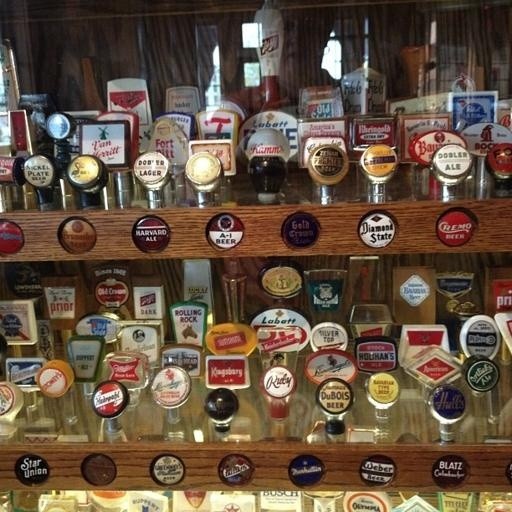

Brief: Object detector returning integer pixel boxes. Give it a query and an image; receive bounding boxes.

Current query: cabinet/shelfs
[0,0,512,512]
